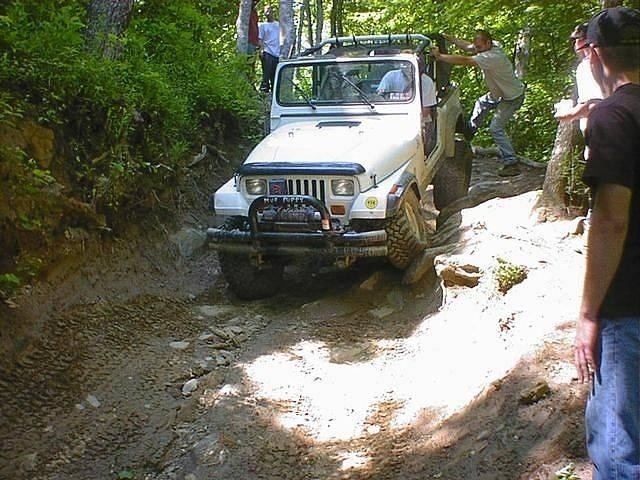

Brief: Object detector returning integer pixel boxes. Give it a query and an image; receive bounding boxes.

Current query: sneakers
[498,163,521,177]
[459,121,473,141]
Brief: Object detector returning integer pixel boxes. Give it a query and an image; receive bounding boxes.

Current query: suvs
[204,28,477,302]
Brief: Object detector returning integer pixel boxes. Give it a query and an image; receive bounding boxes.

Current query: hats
[570,21,589,41]
[576,5,640,51]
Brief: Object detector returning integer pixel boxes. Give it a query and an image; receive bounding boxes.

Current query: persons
[258,5,280,93]
[376,49,437,123]
[554,23,603,254]
[430,30,525,177]
[236,0,259,79]
[573,6,640,480]
